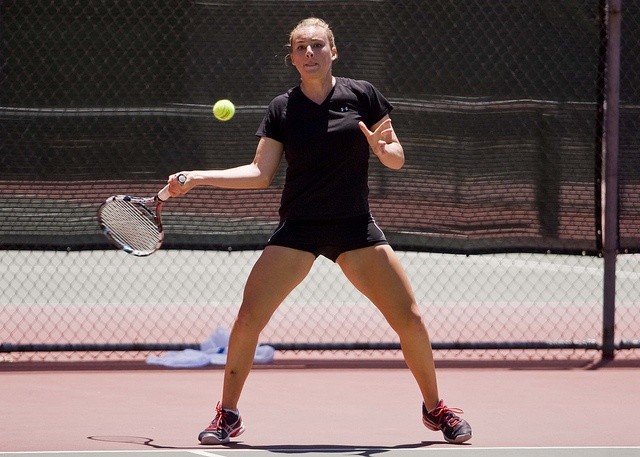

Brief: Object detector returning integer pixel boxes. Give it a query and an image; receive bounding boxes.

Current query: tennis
[213,99,234,119]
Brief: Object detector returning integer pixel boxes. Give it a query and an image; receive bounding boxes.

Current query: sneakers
[198,401,245,444]
[422,399,473,443]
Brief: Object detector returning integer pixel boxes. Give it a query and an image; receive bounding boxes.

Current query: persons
[168,18,472,444]
